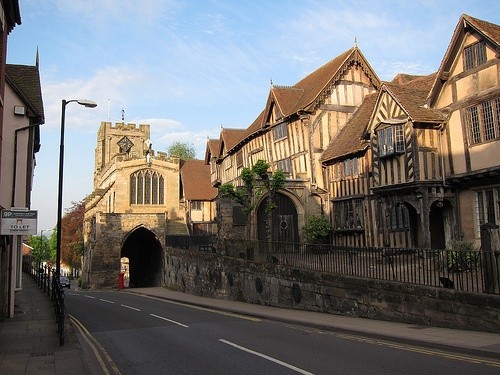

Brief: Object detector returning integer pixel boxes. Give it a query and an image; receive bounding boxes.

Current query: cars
[59,277,70,289]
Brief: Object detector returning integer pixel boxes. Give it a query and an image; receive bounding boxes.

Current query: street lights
[40,230,44,264]
[58,99,97,348]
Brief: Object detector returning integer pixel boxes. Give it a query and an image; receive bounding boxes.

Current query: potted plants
[299,215,331,254]
